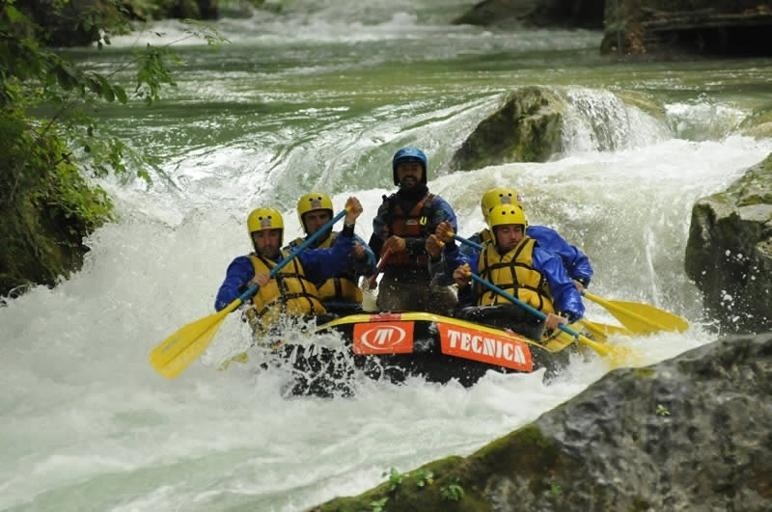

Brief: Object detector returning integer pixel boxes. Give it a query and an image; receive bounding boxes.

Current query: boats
[260,309,594,402]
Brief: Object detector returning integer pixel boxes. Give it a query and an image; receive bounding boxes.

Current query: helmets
[393,148,427,186]
[298,192,334,234]
[481,187,524,226]
[487,204,527,248]
[248,207,284,255]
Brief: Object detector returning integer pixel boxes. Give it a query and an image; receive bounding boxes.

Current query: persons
[359,146,459,316]
[424,186,593,316]
[215,196,362,361]
[444,203,585,345]
[283,193,376,320]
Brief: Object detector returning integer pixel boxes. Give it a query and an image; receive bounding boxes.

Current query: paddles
[149,204,356,379]
[462,264,655,372]
[447,232,689,336]
[577,319,633,342]
